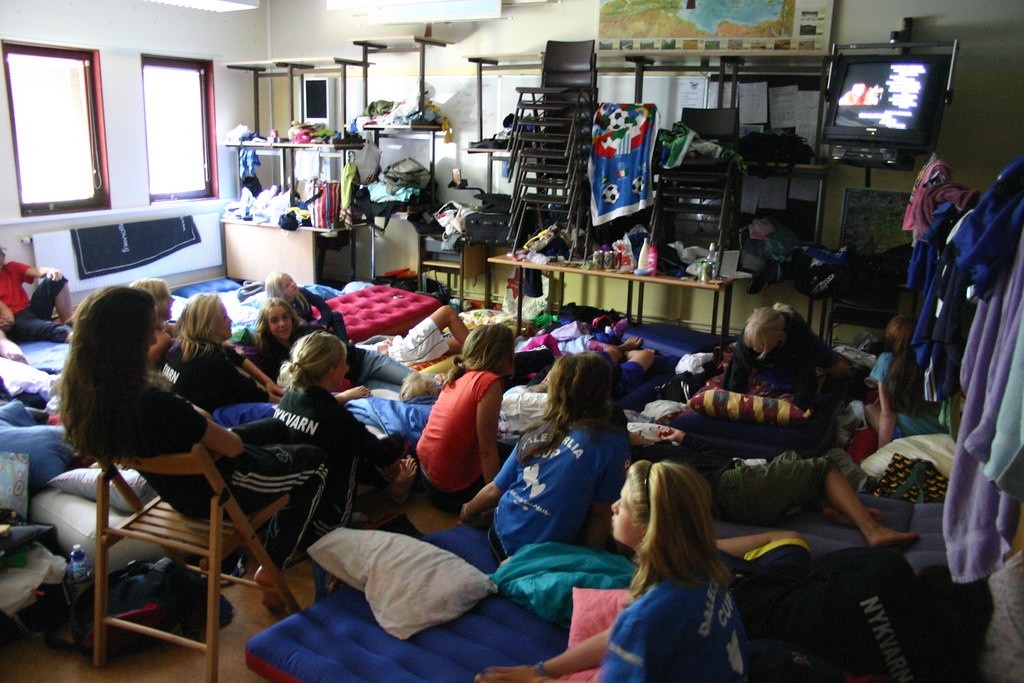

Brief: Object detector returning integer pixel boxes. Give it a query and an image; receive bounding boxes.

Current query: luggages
[464,210,513,248]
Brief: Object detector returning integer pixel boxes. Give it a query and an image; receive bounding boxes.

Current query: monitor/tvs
[821,54,952,147]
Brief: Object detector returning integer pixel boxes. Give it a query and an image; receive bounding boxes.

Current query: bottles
[648,240,657,276]
[637,238,648,271]
[708,243,717,279]
[67,544,92,600]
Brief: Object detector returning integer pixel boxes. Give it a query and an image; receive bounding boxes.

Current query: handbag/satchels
[358,158,438,234]
[42,558,207,663]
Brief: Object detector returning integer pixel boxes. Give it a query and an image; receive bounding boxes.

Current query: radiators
[32,212,223,293]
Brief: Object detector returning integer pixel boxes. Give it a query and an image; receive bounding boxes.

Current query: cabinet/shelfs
[467,50,902,336]
[220,141,364,288]
[417,232,488,312]
[364,120,442,216]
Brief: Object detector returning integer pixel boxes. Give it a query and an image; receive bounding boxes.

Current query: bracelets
[534,661,545,675]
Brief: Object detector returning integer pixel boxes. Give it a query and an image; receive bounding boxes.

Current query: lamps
[144,0,260,13]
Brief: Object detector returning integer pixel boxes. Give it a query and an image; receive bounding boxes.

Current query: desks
[486,253,736,335]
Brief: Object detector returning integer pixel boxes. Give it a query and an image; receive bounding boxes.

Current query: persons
[864,313,950,449]
[125,272,654,567]
[627,427,920,549]
[722,303,847,412]
[474,458,750,683]
[713,531,991,683]
[59,286,329,612]
[0,249,75,346]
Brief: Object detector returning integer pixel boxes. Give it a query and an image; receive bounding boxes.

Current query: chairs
[93,444,302,682]
[824,283,899,348]
[507,39,741,278]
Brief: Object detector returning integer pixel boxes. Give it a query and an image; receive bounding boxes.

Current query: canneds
[593,250,622,269]
[697,260,713,282]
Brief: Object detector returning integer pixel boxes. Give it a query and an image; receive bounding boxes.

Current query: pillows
[0,357,957,683]
